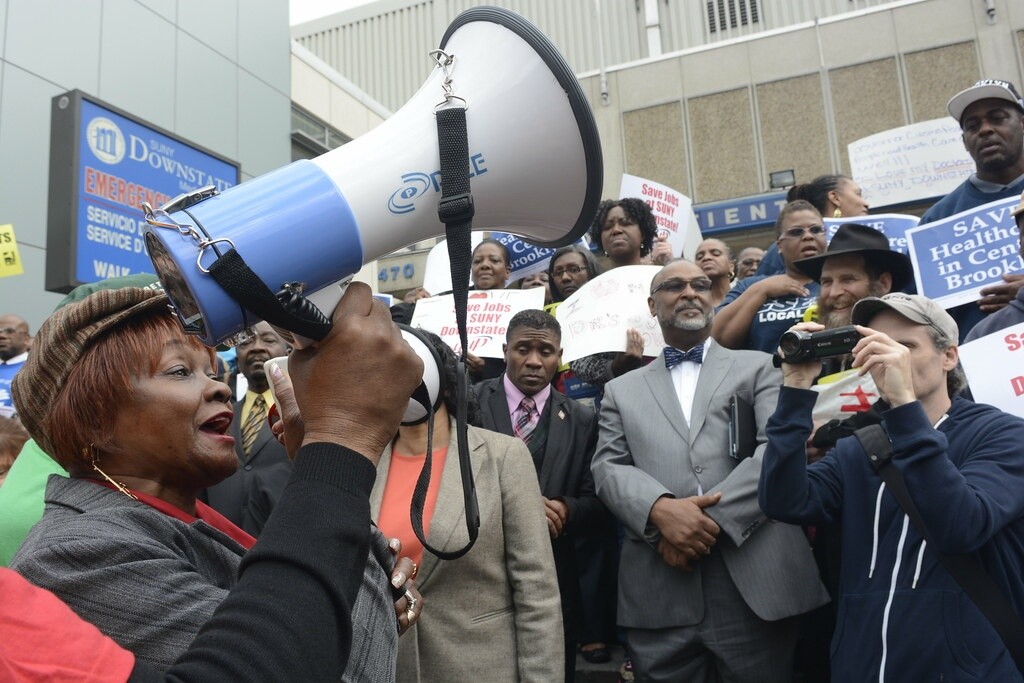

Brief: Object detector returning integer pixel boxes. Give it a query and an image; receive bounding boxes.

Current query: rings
[412,563,417,578]
[405,609,416,627]
[403,588,418,612]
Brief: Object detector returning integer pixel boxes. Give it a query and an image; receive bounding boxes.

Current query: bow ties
[663,344,703,370]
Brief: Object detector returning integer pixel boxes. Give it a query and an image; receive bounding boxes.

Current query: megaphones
[140,6,604,348]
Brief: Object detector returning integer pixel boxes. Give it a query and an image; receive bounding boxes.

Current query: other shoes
[618,654,635,683]
[577,640,611,663]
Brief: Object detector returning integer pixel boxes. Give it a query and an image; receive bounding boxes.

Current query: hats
[850,291,960,347]
[11,272,174,470]
[793,223,913,293]
[1009,189,1024,218]
[946,79,1024,129]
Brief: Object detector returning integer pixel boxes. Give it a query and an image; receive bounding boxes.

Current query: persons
[7,272,423,683]
[758,292,1024,683]
[370,327,565,683]
[785,173,870,217]
[464,238,511,384]
[905,79,1024,347]
[468,309,600,683]
[549,244,602,303]
[591,260,831,683]
[591,197,673,272]
[0,272,425,683]
[0,79,1024,683]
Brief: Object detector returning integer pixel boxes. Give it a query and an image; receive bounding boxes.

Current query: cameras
[778,325,861,361]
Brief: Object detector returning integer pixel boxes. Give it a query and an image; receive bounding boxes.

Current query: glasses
[736,258,761,266]
[653,279,712,292]
[551,266,587,280]
[776,224,827,243]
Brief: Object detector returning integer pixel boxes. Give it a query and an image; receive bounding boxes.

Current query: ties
[514,397,538,447]
[241,395,268,459]
